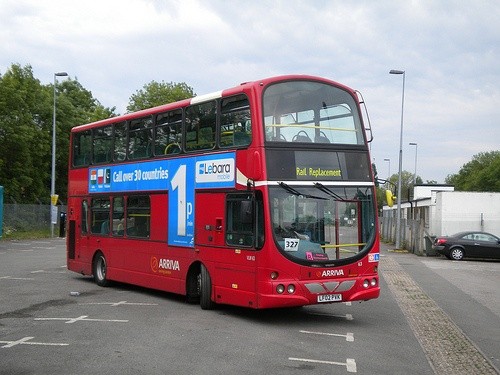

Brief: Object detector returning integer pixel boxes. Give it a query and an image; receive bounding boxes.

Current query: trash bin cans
[424,236,441,257]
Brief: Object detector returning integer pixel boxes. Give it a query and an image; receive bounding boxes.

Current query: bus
[65,74,397,311]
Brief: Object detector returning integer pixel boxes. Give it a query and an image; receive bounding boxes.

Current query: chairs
[84,124,331,164]
[92,218,146,237]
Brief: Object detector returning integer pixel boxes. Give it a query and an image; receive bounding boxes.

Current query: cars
[432,230,500,260]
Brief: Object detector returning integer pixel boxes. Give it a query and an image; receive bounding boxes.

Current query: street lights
[384,158,391,190]
[389,69,406,250]
[49,72,68,238]
[408,142,417,184]
